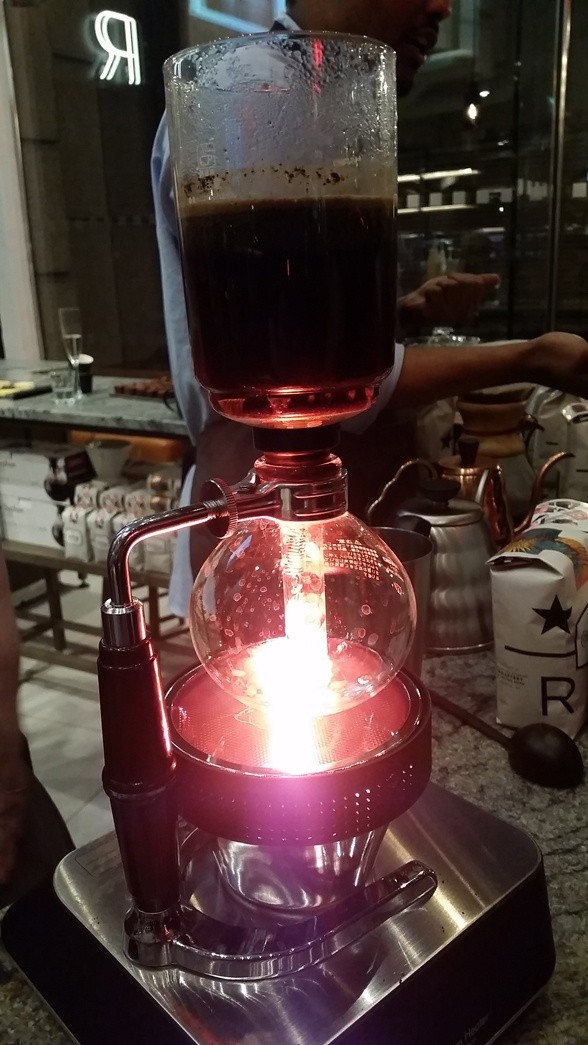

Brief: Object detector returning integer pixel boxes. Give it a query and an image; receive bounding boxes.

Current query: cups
[46,369,77,406]
[86,440,133,487]
[372,527,436,680]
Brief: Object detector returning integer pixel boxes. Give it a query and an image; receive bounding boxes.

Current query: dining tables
[0,374,191,651]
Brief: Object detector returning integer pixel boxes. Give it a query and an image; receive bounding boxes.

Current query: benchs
[0,536,172,675]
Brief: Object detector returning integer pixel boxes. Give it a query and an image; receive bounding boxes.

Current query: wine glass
[58,307,83,401]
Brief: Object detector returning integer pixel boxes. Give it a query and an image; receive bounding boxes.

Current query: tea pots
[365,438,576,658]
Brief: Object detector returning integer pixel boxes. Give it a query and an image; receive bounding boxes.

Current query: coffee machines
[0,30,557,1045]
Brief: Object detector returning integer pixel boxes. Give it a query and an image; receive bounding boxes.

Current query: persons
[154,0,588,632]
